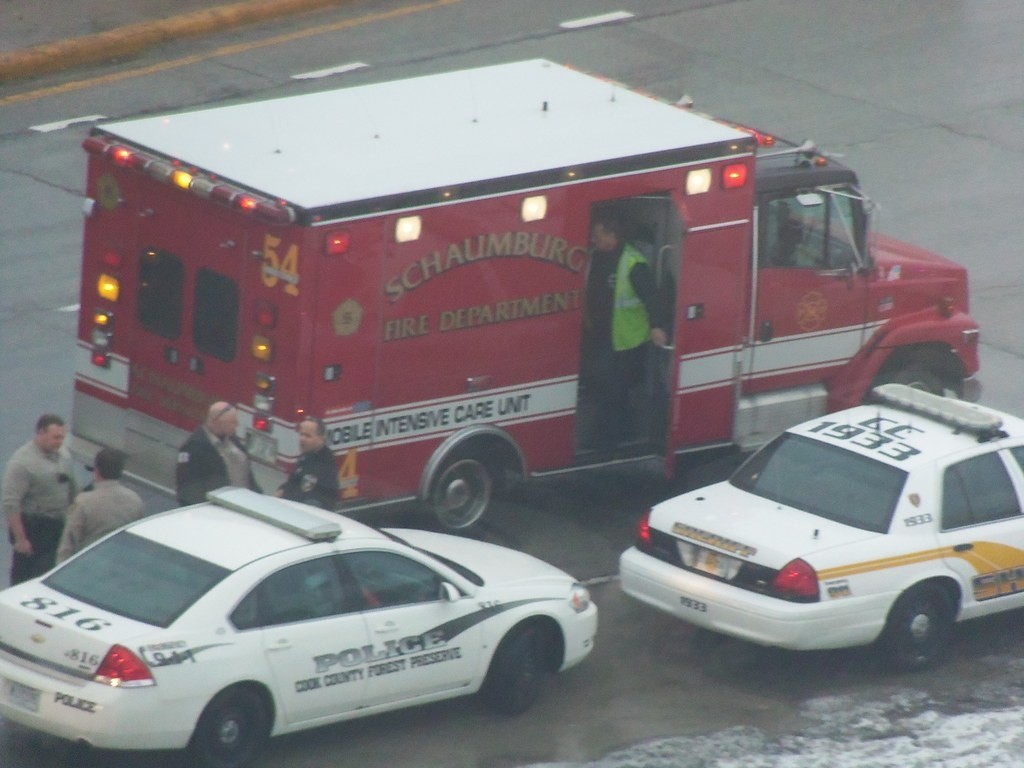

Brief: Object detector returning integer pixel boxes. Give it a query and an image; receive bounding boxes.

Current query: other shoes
[582,436,615,452]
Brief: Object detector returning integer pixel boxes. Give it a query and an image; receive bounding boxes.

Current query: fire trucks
[70,57,987,541]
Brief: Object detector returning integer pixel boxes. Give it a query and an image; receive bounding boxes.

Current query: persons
[277,416,341,515]
[2,415,78,585]
[581,219,669,459]
[174,400,264,508]
[55,447,147,569]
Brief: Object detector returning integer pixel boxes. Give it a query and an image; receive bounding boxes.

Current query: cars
[619,383,1024,676]
[0,485,600,768]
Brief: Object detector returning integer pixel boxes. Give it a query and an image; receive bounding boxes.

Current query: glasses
[214,403,232,419]
[305,415,321,424]
